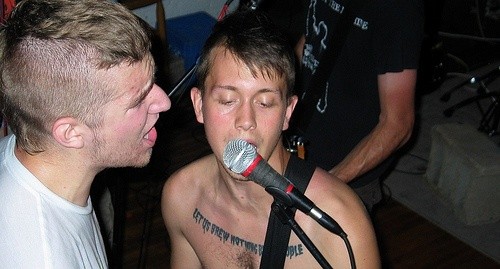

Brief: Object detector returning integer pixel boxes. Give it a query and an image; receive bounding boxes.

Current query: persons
[160,10,382,268]
[1,0,171,268]
[290,0,421,217]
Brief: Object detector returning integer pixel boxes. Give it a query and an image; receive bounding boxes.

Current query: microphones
[224,140,349,239]
